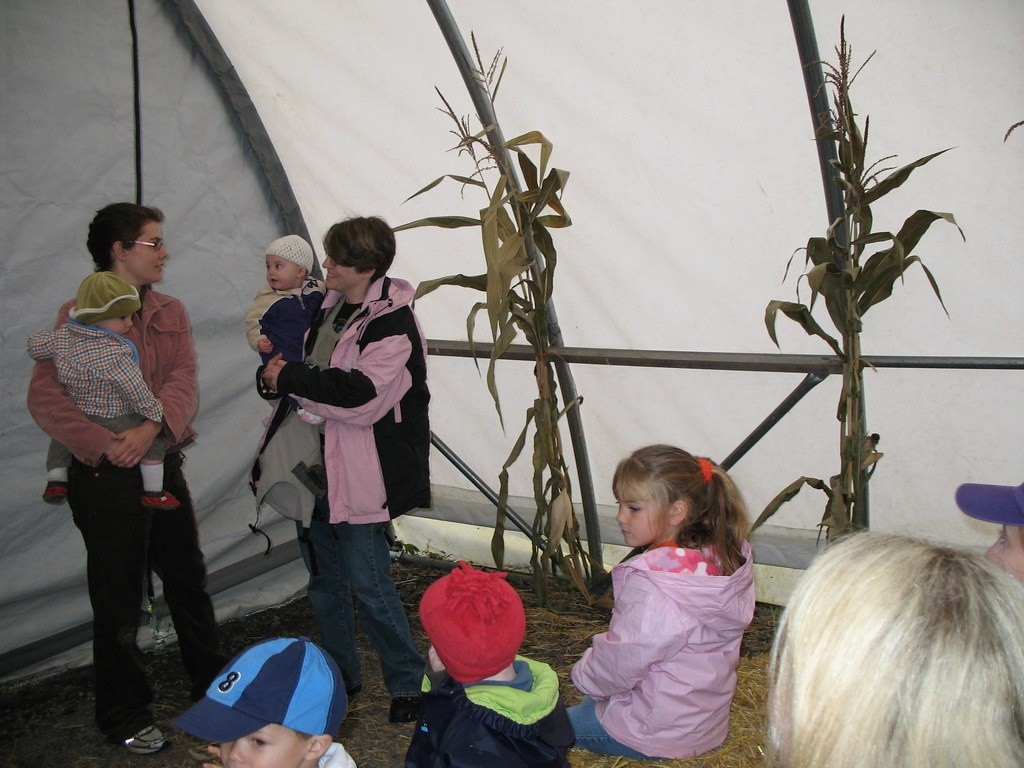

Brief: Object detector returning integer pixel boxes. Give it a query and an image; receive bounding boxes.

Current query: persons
[174,637,358,768]
[246,216,431,722]
[768,479,1024,768]
[29,270,181,508]
[28,203,220,755]
[565,445,756,760]
[246,235,330,429]
[406,560,578,768]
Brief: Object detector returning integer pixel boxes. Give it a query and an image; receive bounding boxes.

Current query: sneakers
[123,725,166,755]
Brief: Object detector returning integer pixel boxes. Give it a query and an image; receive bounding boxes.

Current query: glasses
[130,240,166,251]
[69,270,142,325]
[324,247,342,267]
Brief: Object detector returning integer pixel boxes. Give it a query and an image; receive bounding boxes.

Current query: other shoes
[388,695,424,729]
[263,416,268,428]
[140,488,181,511]
[42,480,69,506]
[297,405,326,425]
[348,692,361,705]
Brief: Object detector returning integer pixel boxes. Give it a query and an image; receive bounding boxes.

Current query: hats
[955,482,1024,527]
[417,560,525,684]
[265,234,314,276]
[173,637,349,744]
